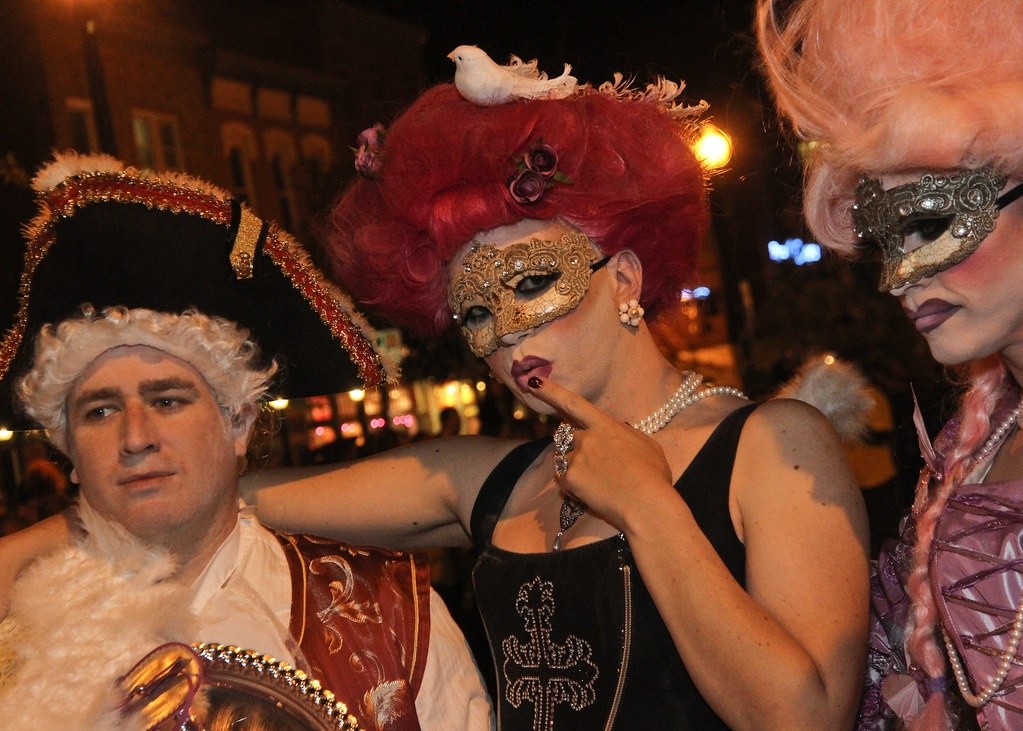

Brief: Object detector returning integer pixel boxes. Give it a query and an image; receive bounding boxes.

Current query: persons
[0,220,872,731]
[439,407,461,437]
[0,345,492,731]
[853,168,1023,731]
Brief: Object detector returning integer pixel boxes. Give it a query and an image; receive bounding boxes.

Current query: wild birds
[448,45,577,105]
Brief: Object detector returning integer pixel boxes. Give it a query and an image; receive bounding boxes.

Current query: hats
[0,152,404,433]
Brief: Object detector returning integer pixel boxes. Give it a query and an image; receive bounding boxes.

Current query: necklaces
[552,370,748,553]
[940,401,1023,706]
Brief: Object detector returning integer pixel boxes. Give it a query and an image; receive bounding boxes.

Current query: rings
[553,454,567,477]
[552,423,574,452]
